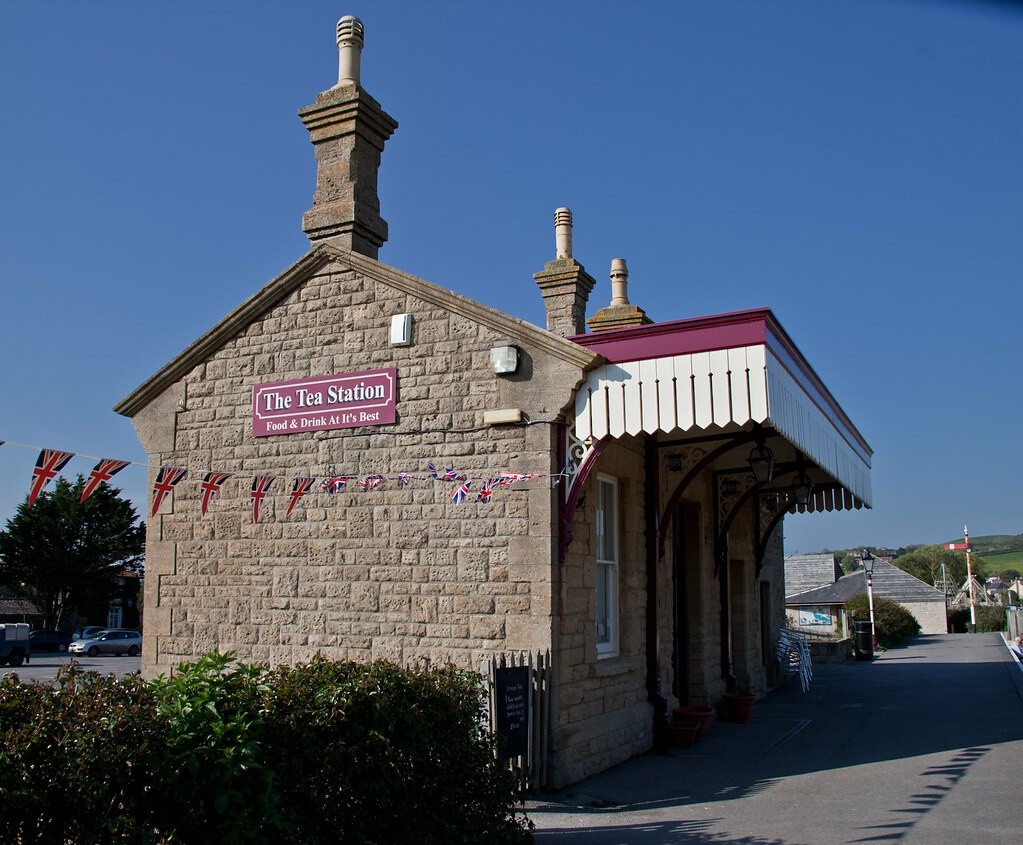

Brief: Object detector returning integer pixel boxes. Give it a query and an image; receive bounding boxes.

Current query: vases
[670,705,714,749]
[722,694,755,724]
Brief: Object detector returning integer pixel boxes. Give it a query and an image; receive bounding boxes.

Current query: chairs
[775,628,813,694]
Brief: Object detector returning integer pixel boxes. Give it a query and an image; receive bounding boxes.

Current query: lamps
[489,345,522,376]
[789,470,814,505]
[746,440,777,484]
[666,455,680,472]
[726,481,737,495]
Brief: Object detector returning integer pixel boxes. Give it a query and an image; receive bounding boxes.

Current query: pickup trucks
[0,623,29,668]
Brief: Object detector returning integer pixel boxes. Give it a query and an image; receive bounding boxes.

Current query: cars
[69,630,142,657]
[71,626,104,641]
[29,630,69,653]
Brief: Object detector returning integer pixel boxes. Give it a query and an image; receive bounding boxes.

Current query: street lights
[964,524,977,634]
[861,547,876,652]
